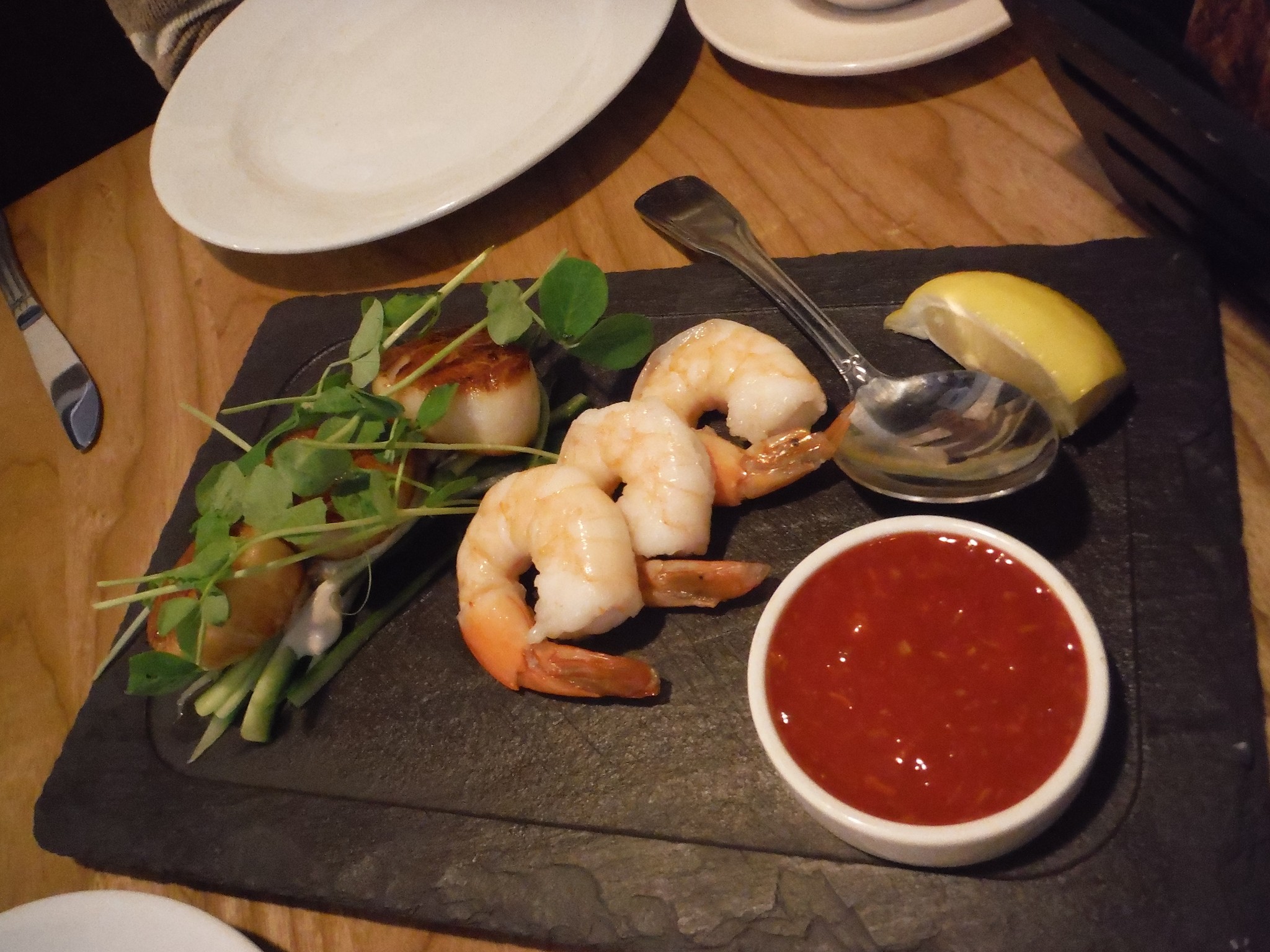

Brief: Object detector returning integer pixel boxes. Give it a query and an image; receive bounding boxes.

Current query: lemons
[882,270,1125,436]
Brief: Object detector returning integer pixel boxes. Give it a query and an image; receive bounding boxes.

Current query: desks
[0,31,1269,952]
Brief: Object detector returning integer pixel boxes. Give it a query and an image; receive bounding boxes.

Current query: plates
[1,890,261,952]
[29,232,1269,950]
[685,0,1017,79]
[148,0,679,253]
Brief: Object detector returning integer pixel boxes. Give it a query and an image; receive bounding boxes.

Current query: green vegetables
[91,245,654,769]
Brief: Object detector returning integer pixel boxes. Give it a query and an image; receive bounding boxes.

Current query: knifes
[0,214,101,449]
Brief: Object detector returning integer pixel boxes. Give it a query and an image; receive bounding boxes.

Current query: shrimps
[628,318,859,507]
[455,463,667,700]
[549,401,773,610]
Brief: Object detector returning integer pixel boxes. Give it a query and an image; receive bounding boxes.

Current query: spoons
[636,174,1059,503]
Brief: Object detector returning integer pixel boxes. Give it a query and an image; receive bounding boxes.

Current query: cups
[746,515,1111,868]
